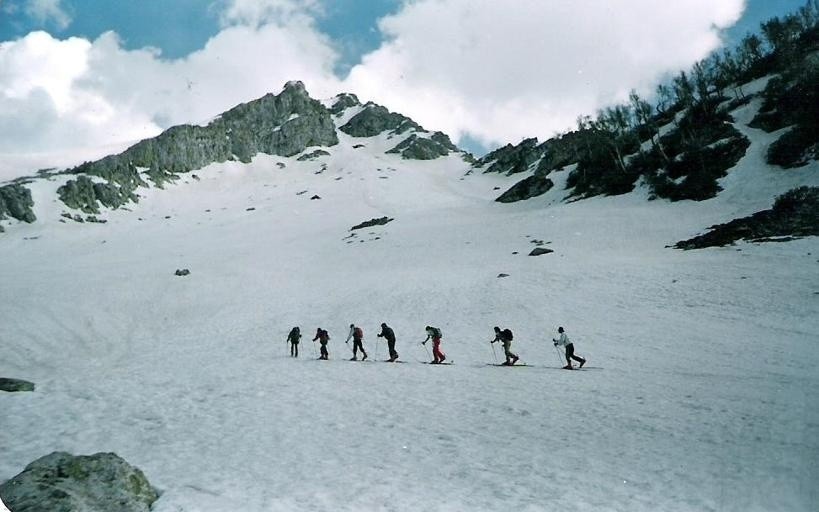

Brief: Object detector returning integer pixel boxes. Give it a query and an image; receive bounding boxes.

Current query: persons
[491,325,519,366]
[422,326,447,363]
[313,328,330,360]
[286,327,302,357]
[552,327,586,370]
[377,322,399,362]
[344,324,367,362]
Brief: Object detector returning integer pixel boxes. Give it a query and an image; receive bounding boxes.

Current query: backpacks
[355,328,363,337]
[500,329,512,340]
[431,328,442,338]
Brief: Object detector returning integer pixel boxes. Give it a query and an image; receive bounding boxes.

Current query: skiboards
[419,358,457,365]
[544,364,603,372]
[487,362,533,368]
[340,357,373,362]
[375,358,408,365]
